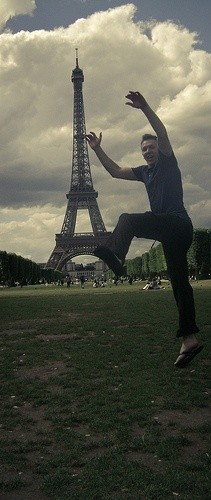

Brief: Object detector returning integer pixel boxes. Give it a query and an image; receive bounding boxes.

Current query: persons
[83,90,205,370]
[3,273,201,291]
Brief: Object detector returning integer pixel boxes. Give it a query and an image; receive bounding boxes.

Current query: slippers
[92,246,126,278]
[174,343,204,367]
